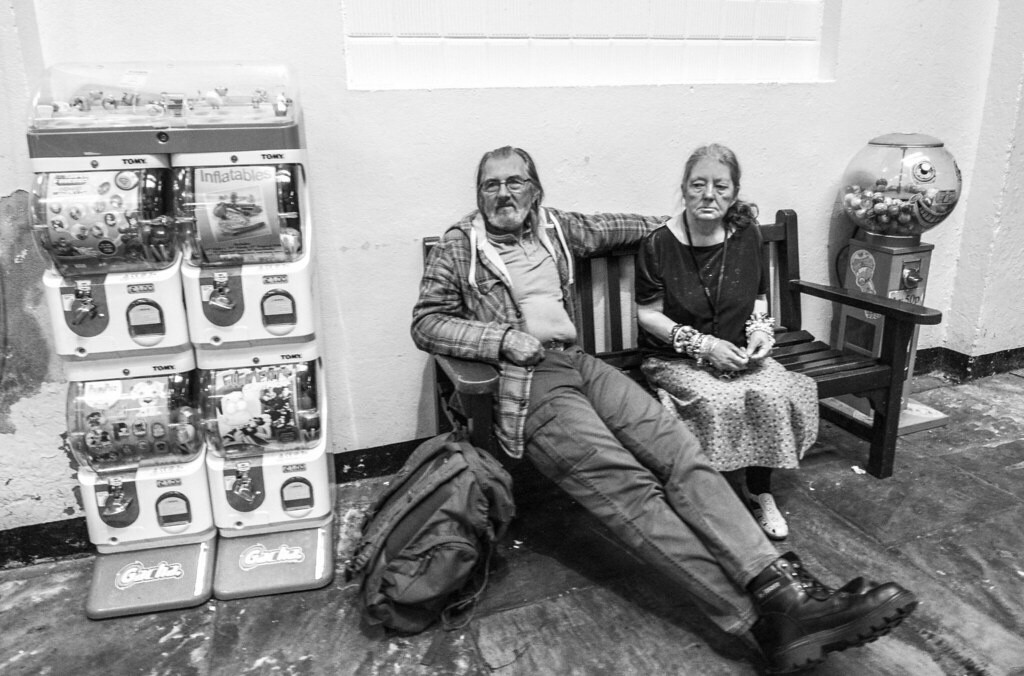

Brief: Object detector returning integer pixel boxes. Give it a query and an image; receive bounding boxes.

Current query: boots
[751,555,916,673]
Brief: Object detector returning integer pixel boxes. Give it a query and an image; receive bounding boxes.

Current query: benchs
[422,208,943,481]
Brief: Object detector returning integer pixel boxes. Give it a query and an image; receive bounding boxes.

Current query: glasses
[476,175,530,193]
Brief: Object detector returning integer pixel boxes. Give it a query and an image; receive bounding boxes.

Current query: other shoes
[752,493,789,543]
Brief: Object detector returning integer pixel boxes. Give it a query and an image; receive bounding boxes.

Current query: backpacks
[351,432,516,635]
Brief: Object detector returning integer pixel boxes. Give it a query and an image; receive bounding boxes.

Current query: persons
[630,145,820,538]
[408,145,921,676]
